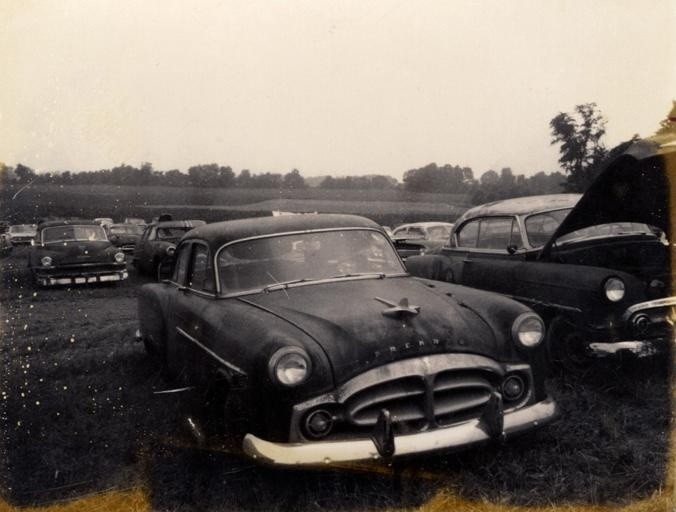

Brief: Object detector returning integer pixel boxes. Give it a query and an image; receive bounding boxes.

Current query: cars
[406,126,676,380]
[132,208,565,481]
